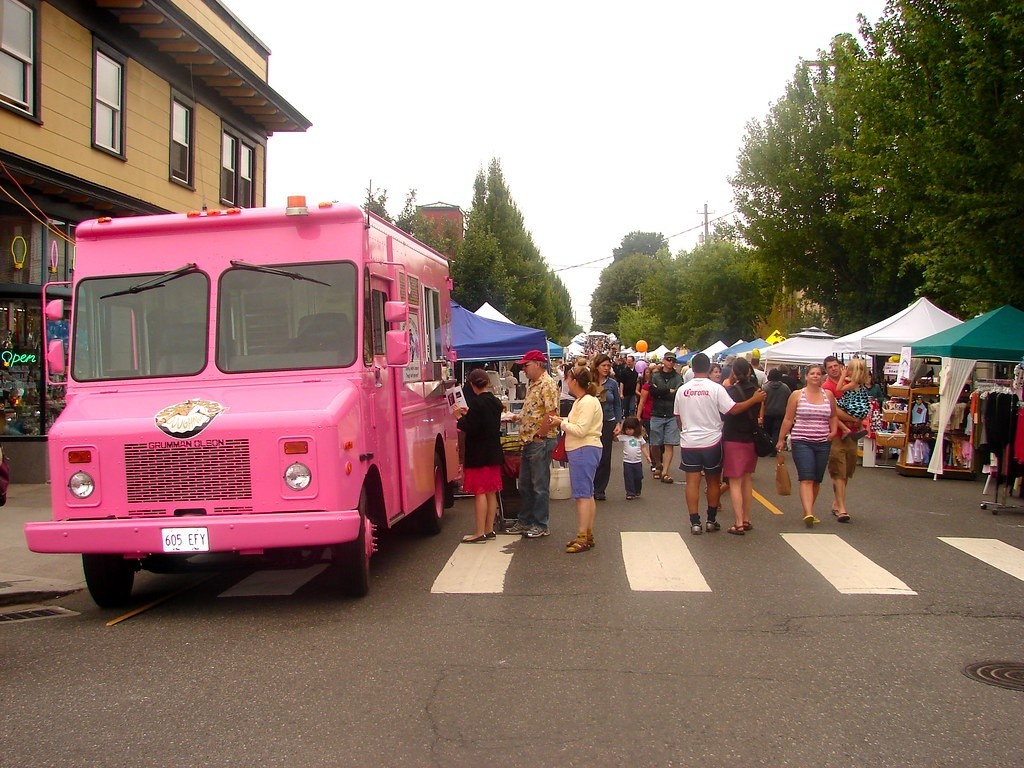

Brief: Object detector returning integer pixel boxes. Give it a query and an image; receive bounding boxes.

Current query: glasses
[666,358,676,362]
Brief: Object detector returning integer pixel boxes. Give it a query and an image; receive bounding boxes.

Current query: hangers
[969,379,1014,399]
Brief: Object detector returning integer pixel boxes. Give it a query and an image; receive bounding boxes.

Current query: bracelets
[535,433,543,439]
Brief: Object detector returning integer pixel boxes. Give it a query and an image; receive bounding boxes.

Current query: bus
[21,193,462,609]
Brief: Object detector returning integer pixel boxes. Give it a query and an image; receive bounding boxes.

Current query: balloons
[636,339,648,352]
[635,361,647,374]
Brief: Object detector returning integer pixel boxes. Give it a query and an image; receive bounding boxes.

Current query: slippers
[832,507,851,521]
[803,516,821,528]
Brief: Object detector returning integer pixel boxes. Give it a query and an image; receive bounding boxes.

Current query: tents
[592,298,1024,495]
[450,297,588,389]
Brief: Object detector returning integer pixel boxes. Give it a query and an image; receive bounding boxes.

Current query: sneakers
[526,524,550,537]
[505,520,532,534]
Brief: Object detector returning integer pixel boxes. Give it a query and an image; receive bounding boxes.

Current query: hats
[574,358,591,368]
[664,352,677,360]
[517,350,546,364]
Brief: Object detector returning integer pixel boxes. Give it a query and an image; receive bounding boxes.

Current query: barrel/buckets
[548,467,572,500]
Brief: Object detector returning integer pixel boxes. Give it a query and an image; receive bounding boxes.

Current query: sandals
[727,523,745,535]
[661,472,674,483]
[745,520,753,531]
[654,466,662,479]
[563,536,597,553]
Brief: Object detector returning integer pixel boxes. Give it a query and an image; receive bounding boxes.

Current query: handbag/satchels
[502,455,521,478]
[775,450,791,495]
[753,425,774,457]
[550,431,568,462]
[0,456,10,507]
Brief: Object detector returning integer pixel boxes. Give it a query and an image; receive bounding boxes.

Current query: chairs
[297,311,352,367]
[155,322,207,375]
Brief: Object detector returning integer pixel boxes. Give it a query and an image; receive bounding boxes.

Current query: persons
[453,369,505,544]
[506,350,560,537]
[548,366,603,552]
[586,337,881,534]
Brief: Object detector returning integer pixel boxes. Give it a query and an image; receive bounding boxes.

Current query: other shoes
[461,530,487,544]
[855,429,868,438]
[486,531,496,541]
[841,430,851,440]
[594,492,606,500]
[706,520,720,532]
[626,492,640,500]
[691,523,702,534]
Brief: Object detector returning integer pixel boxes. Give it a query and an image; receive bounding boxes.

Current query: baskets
[886,384,911,398]
[881,407,908,423]
[876,431,907,447]
